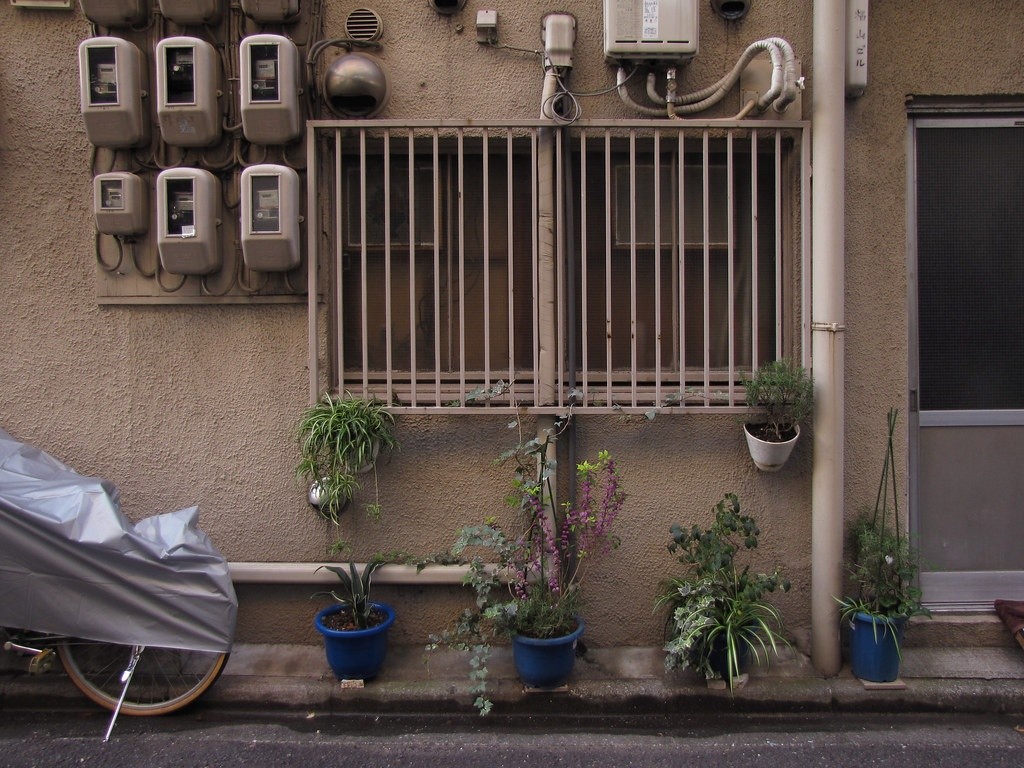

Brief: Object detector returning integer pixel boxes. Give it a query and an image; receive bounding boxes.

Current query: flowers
[415,380,631,717]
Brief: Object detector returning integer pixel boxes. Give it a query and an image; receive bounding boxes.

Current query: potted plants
[650,492,797,699]
[310,558,396,680]
[293,390,403,559]
[739,358,817,472]
[830,508,932,680]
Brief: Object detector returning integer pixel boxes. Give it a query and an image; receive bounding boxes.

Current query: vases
[508,610,584,688]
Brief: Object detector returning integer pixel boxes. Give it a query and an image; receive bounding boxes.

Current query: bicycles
[0,627,231,744]
